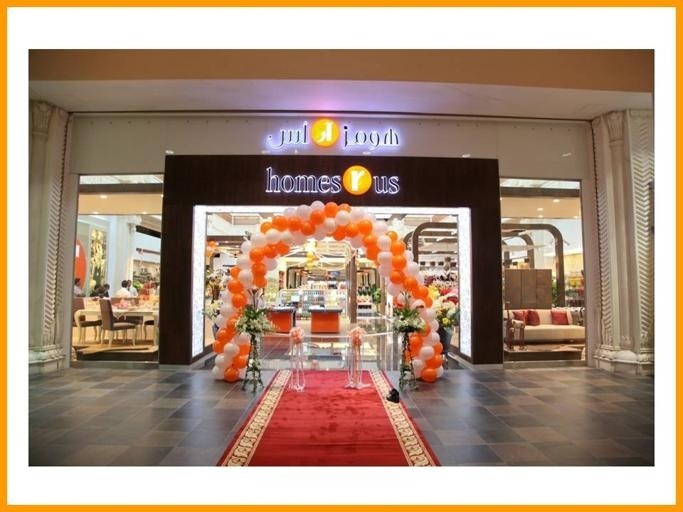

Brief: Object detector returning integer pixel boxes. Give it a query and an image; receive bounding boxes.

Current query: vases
[211,322,221,337]
[438,325,455,359]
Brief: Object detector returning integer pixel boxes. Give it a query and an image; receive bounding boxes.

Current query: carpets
[503,340,587,354]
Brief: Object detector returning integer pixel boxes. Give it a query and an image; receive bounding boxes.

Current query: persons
[73,276,161,300]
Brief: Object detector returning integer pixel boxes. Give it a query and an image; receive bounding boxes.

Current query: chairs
[72,296,159,347]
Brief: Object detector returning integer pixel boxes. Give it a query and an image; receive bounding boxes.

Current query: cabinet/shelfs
[504,269,537,309]
[565,272,585,307]
[537,269,552,309]
[280,281,376,319]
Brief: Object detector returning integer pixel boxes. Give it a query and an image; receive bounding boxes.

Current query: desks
[265,306,295,333]
[308,304,342,334]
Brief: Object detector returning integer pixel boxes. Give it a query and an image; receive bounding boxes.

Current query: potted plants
[373,287,386,314]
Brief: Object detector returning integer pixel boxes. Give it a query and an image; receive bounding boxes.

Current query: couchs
[503,306,588,349]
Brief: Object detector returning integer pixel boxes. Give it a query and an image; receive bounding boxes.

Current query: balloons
[209,201,444,383]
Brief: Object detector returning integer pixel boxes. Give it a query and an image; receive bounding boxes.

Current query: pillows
[512,307,583,325]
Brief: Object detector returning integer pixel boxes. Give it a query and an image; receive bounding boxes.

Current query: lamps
[139,248,144,256]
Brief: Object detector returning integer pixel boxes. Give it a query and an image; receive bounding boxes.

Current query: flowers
[233,288,280,334]
[424,271,461,325]
[389,292,426,330]
[204,269,224,320]
